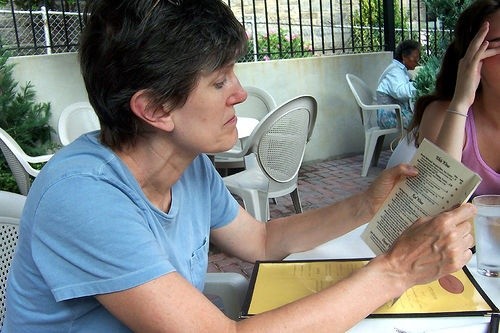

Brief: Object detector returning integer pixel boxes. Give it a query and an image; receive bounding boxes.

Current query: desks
[281,220,500,333]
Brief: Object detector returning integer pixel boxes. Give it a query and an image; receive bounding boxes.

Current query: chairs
[345,71,405,177]
[213,86,278,177]
[0,188,249,333]
[0,125,55,196]
[204,93,320,222]
[58,102,102,147]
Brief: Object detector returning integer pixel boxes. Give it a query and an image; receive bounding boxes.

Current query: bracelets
[445,108,468,117]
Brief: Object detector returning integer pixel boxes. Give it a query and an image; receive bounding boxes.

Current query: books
[360,138,482,310]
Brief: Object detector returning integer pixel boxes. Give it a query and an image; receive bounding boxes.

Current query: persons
[418,0,500,204]
[0,0,475,333]
[377,40,421,129]
[386,95,434,168]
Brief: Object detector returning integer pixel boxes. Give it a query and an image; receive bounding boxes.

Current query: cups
[474,193,500,277]
